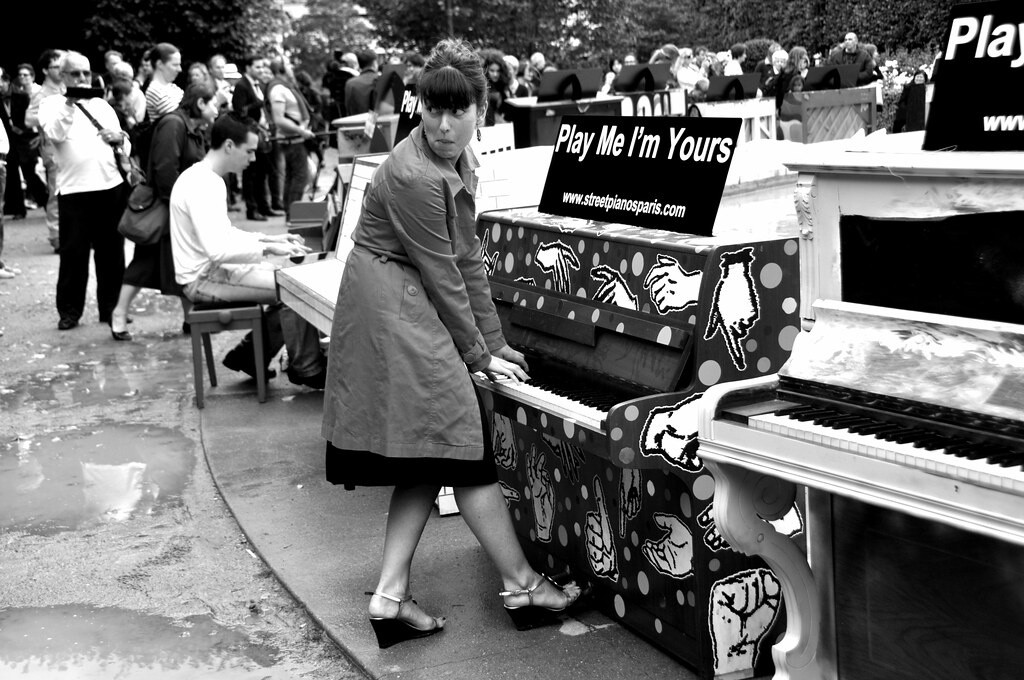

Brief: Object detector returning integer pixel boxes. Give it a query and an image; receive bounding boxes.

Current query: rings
[108,135,111,137]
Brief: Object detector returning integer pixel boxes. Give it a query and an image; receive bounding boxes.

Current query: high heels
[500,573,583,631]
[364,589,445,648]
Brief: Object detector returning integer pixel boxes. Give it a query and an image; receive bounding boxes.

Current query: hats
[222,64,242,79]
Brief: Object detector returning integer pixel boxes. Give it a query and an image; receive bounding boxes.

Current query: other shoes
[272,200,285,211]
[247,209,269,221]
[259,206,281,216]
[99,313,135,324]
[287,366,330,389]
[58,316,77,330]
[0,263,21,278]
[110,311,131,340]
[183,320,191,334]
[223,346,276,379]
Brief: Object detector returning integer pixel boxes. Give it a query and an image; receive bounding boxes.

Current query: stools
[185,302,268,410]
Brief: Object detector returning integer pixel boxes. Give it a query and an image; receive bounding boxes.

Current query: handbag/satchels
[118,183,167,245]
[115,149,146,188]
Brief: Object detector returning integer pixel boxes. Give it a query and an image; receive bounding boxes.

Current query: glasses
[60,68,92,80]
[19,72,33,78]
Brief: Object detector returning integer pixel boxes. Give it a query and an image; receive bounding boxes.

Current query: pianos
[289,86,878,359]
[272,153,542,518]
[463,204,801,680]
[696,125,1024,680]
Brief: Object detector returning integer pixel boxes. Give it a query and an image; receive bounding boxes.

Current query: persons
[319,39,590,647]
[170,111,329,388]
[0,31,928,340]
